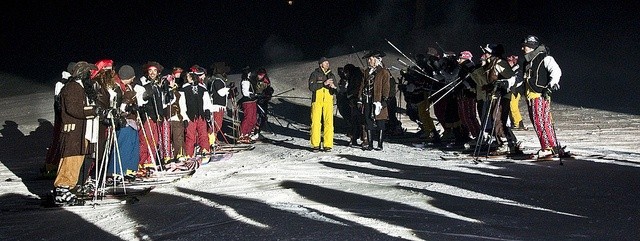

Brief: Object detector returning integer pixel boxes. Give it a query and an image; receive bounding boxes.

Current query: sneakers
[509,142,519,151]
[324,148,331,152]
[464,137,484,147]
[496,144,511,152]
[313,146,320,152]
[531,149,553,159]
[55,187,79,204]
[511,122,524,128]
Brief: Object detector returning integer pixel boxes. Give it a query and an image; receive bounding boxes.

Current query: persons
[359,50,391,151]
[88,60,235,185]
[240,66,257,141]
[51,62,89,207]
[54,63,75,97]
[309,56,340,153]
[252,67,271,123]
[505,54,523,130]
[484,43,520,155]
[521,35,563,159]
[398,48,483,145]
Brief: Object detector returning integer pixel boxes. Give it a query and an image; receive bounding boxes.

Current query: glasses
[522,37,528,44]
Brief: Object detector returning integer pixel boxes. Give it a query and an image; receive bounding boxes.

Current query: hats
[521,35,540,49]
[506,55,519,65]
[319,56,330,64]
[485,43,505,57]
[67,62,76,75]
[362,48,386,59]
[72,61,98,79]
[460,51,473,59]
[118,65,135,80]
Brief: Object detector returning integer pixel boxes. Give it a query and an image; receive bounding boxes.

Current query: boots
[362,129,373,150]
[375,130,383,150]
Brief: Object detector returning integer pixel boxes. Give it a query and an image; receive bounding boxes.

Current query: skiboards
[440,151,526,163]
[222,132,293,145]
[474,150,604,164]
[20,140,255,210]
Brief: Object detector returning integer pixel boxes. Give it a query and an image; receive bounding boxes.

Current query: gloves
[541,84,555,100]
[493,80,509,91]
[95,107,117,125]
[125,103,145,118]
